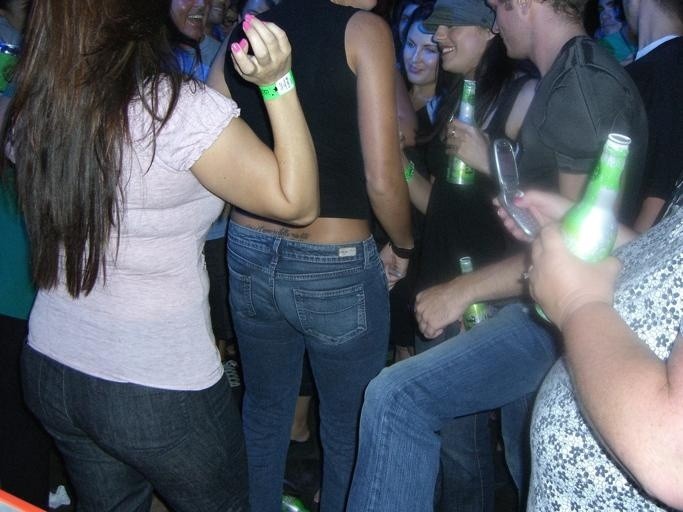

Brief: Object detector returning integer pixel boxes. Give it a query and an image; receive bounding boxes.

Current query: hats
[421,0,497,30]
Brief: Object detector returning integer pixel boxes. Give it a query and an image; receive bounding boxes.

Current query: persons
[1,2,51,511]
[1,1,319,512]
[168,1,683,510]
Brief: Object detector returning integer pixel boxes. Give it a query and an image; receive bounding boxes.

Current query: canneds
[0,43,23,92]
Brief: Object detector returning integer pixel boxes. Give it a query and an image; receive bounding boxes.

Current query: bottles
[443,79,480,186]
[459,257,489,326]
[537,132,634,318]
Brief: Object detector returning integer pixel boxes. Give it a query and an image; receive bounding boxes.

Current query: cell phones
[491,136,542,238]
